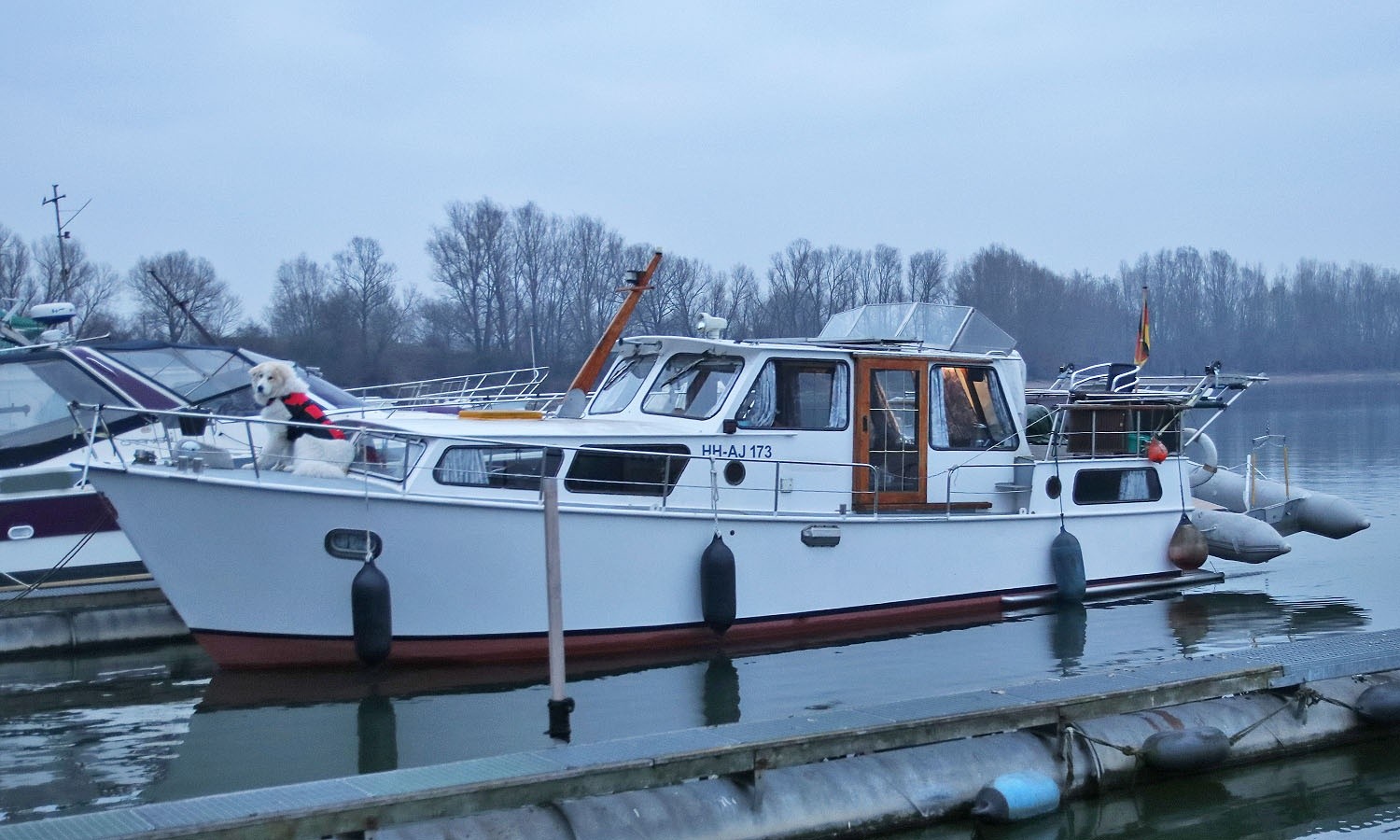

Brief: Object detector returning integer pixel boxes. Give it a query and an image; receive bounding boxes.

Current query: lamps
[696,313,728,339]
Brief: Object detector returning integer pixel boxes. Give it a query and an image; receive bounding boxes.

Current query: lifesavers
[459,410,543,421]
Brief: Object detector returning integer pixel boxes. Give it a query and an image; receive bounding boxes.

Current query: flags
[1133,299,1150,364]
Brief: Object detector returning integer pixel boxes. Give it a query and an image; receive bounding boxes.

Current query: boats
[1,183,386,667]
[66,237,1373,678]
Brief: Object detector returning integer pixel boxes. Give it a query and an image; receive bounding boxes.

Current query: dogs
[248,363,354,476]
